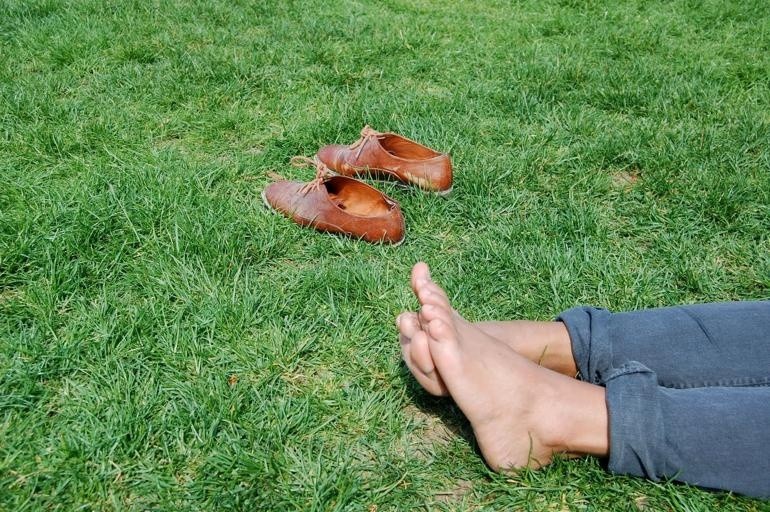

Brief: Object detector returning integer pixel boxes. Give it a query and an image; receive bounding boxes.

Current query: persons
[393,256,770,506]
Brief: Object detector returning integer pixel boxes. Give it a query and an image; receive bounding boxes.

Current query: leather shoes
[261,156,405,248]
[313,124,454,196]
